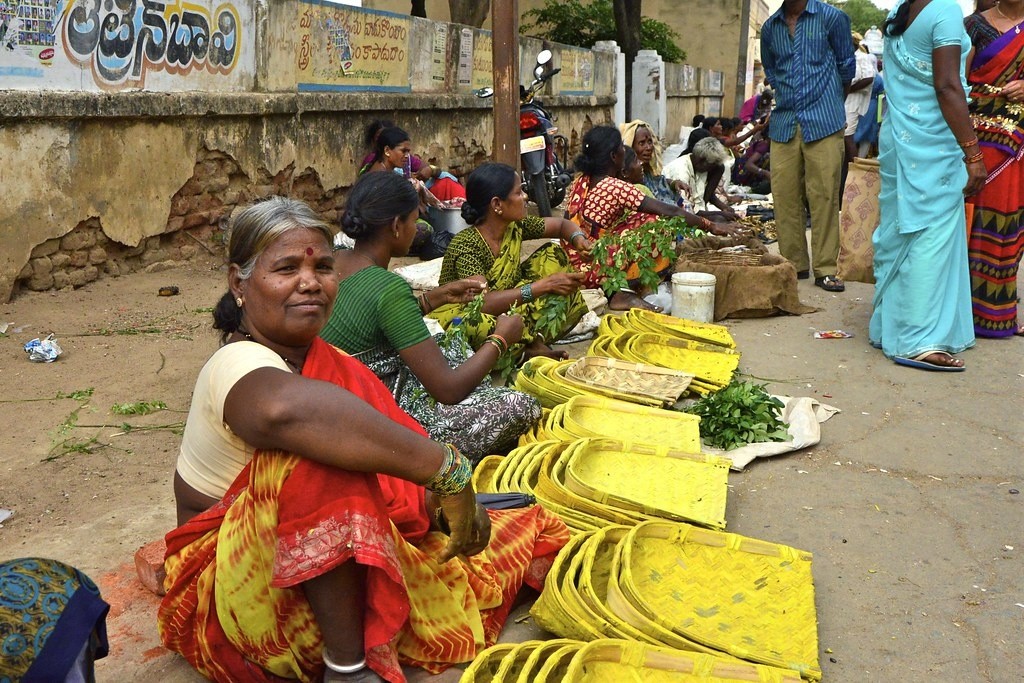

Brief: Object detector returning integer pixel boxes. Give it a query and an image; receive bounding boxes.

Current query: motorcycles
[476,49,572,219]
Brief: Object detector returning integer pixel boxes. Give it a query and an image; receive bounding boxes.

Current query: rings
[1014,97,1018,101]
[743,225,746,228]
[737,229,743,233]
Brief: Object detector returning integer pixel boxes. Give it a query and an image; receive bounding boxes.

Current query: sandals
[815,274,845,292]
[796,270,810,280]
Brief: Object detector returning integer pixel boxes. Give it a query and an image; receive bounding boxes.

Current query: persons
[356,119,466,248]
[756,77,770,94]
[617,119,692,209]
[739,89,774,124]
[316,171,542,462]
[173,198,476,683]
[761,0,857,292]
[838,28,884,210]
[868,0,1024,372]
[438,163,595,387]
[661,115,773,223]
[562,119,756,241]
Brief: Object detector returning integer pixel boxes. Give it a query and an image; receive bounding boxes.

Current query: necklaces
[380,162,387,170]
[996,6,1022,34]
[239,331,302,372]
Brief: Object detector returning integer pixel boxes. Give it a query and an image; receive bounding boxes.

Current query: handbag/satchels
[831,157,884,285]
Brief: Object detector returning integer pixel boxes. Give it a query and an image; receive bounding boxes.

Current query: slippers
[891,349,966,371]
[871,341,883,349]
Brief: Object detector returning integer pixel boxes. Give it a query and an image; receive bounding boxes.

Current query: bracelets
[417,293,433,316]
[763,121,768,126]
[418,443,473,497]
[697,216,711,232]
[480,334,508,359]
[962,151,984,163]
[670,179,673,185]
[958,135,978,147]
[430,165,437,177]
[570,231,587,244]
[520,283,535,304]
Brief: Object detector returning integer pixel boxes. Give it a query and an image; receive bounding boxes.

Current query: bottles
[450,317,467,357]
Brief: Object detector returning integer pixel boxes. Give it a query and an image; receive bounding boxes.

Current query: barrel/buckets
[671,272,717,326]
[427,202,472,235]
